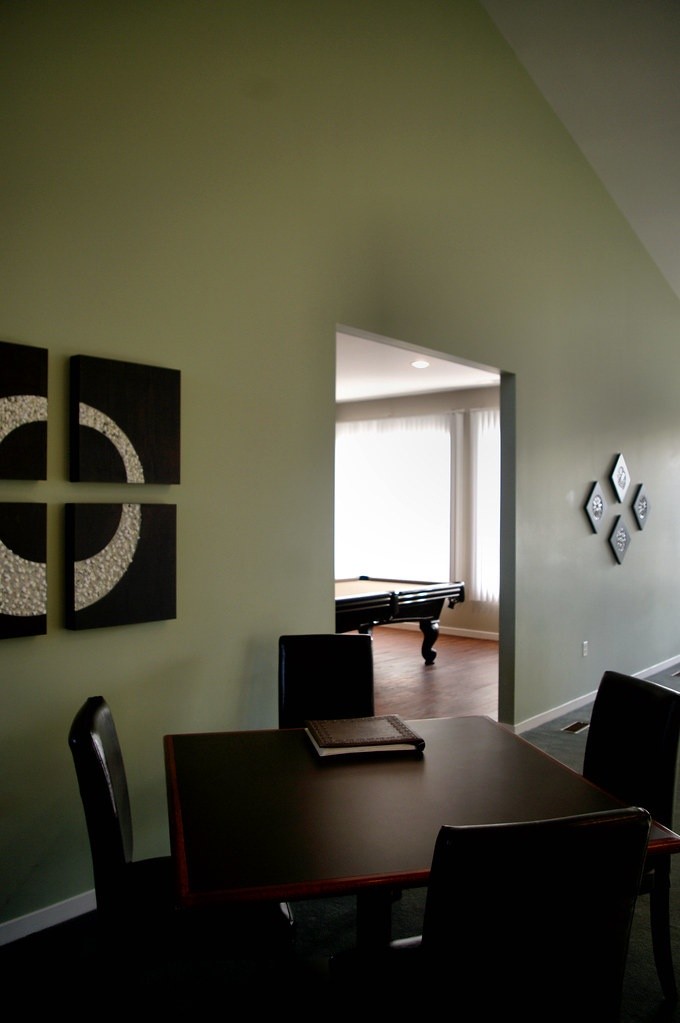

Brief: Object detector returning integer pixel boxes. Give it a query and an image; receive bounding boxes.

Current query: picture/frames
[609,515,630,564]
[583,481,607,534]
[608,453,631,503]
[631,483,652,530]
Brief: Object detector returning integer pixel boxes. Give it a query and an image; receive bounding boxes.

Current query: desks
[332,576,467,668]
[164,715,680,1023]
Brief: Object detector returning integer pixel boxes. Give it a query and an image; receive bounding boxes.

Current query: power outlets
[583,641,589,657]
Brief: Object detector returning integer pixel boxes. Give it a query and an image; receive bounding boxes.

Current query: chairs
[277,635,373,729]
[69,693,296,1023]
[372,806,652,1023]
[582,670,680,1005]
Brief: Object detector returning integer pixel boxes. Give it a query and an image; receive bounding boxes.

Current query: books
[304,716,425,758]
